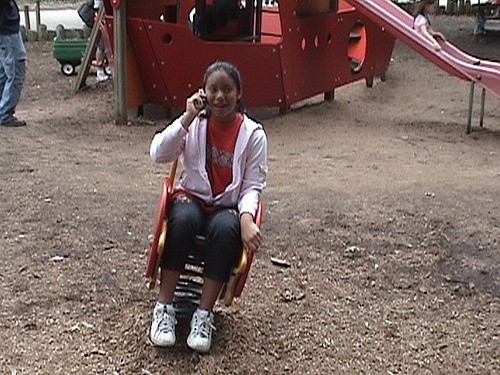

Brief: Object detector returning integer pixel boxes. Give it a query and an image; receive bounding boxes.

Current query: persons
[92,0,109,84]
[150,61,268,352]
[0,0,27,126]
[412,0,480,65]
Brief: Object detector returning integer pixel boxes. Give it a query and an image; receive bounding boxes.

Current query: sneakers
[186,308,217,353]
[150,301,179,347]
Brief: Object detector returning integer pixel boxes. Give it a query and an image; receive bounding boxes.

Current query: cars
[51,24,108,76]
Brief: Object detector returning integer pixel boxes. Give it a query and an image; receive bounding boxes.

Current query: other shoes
[96,68,112,81]
[1,118,27,126]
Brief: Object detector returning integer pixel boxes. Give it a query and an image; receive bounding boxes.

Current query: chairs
[146,159,263,313]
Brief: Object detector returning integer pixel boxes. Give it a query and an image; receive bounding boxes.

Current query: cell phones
[199,88,206,103]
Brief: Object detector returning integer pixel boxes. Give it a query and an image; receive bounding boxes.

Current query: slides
[343,0,500,99]
